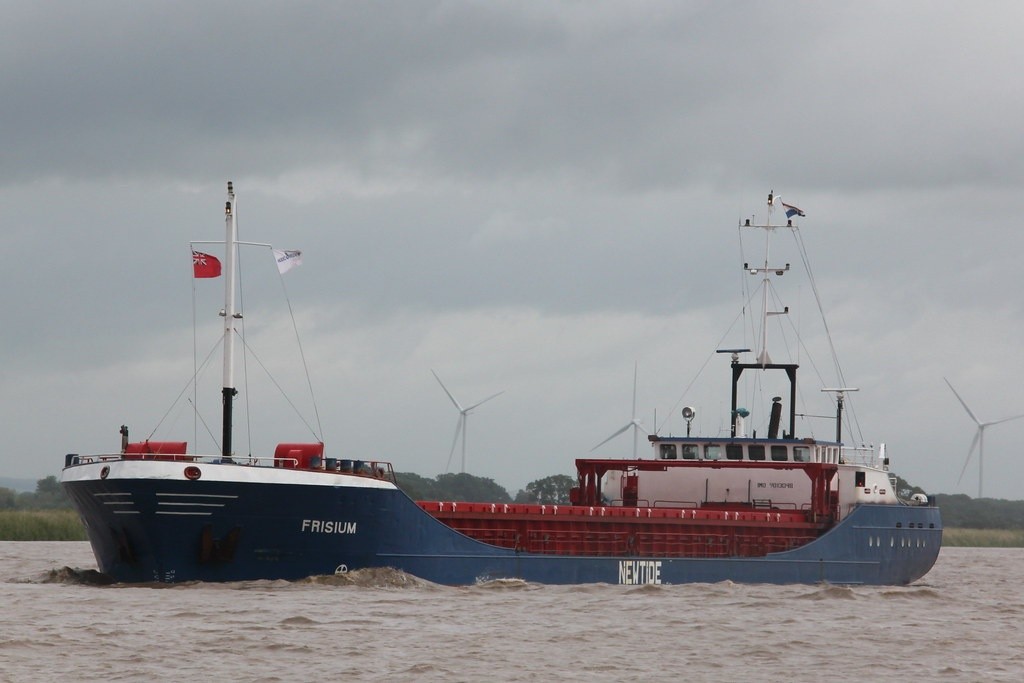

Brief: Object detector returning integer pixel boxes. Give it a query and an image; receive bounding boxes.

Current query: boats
[55,177,942,588]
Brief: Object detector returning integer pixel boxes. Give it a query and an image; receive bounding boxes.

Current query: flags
[193,251,221,278]
[274,249,304,272]
[783,203,805,218]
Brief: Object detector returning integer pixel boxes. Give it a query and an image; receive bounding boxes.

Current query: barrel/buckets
[927,496,935,506]
[64,454,79,466]
[310,456,365,474]
